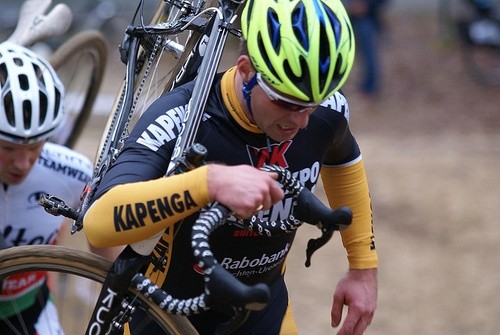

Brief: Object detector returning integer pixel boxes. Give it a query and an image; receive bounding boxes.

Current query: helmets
[241,0,356,104]
[0,40,66,144]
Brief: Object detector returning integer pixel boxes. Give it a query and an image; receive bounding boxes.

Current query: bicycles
[0,0,355,335]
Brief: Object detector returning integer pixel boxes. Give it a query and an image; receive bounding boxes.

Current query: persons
[0,41,95,335]
[83,0,377,335]
[340,0,382,93]
